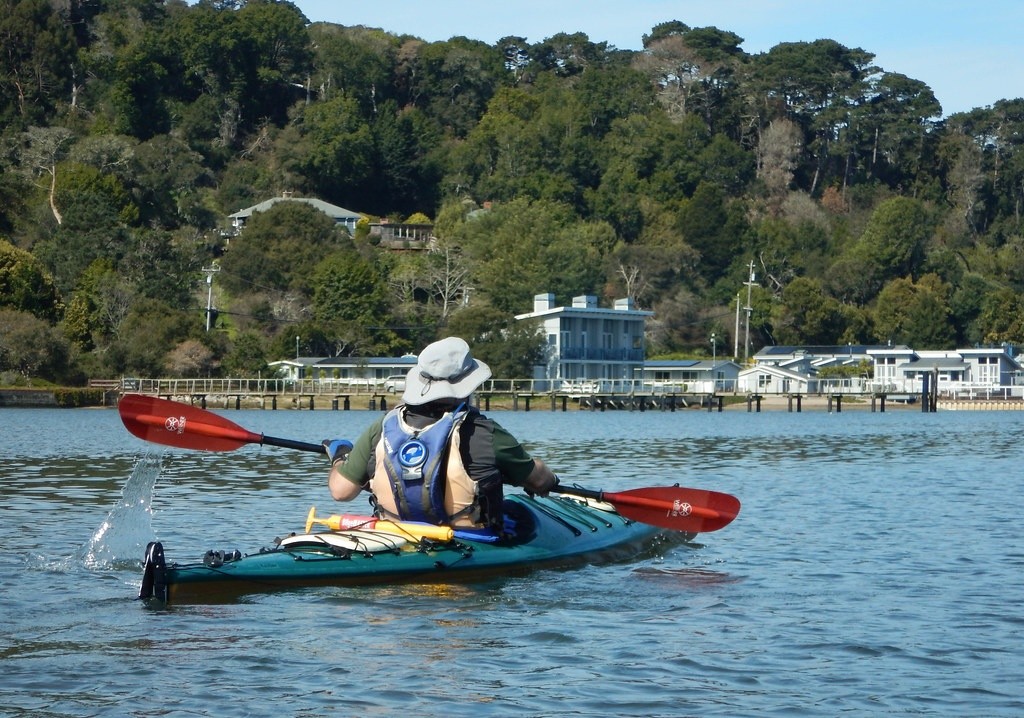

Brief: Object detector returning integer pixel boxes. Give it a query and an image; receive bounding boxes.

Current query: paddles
[117,391,742,534]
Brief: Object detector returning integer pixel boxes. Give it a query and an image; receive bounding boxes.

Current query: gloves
[321,438,355,463]
[525,473,561,500]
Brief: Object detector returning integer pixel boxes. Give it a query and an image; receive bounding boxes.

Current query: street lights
[202,266,222,331]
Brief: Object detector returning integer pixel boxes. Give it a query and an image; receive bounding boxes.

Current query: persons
[322,336,560,528]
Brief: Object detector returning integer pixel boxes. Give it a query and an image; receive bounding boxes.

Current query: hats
[402,336,492,405]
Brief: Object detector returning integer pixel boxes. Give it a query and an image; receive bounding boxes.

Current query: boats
[139,482,705,612]
